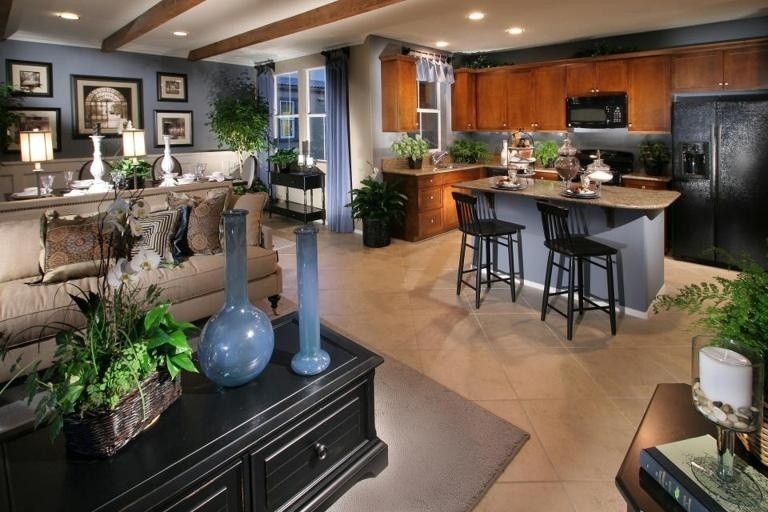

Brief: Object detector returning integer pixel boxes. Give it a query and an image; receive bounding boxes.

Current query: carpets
[321,315,531,511]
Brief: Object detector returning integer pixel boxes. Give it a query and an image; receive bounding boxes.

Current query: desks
[451,178,682,321]
[615,383,768,512]
[271,169,326,225]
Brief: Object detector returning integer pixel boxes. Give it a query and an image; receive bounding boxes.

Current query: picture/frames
[3,58,194,154]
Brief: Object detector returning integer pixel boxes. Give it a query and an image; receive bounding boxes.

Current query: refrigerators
[671,94,768,278]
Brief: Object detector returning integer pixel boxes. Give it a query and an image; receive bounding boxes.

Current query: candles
[699,345,753,410]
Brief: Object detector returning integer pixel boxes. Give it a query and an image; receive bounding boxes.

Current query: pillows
[25,187,269,286]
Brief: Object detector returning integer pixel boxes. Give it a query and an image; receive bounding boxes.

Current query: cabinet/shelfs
[528,172,561,180]
[0,311,389,511]
[379,54,419,133]
[621,178,668,190]
[383,167,489,243]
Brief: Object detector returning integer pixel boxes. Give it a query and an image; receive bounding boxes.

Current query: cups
[508,170,517,183]
[580,174,590,189]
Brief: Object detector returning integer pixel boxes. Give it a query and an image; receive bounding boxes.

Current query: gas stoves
[560,165,633,184]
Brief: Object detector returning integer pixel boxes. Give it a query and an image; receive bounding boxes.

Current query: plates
[12,191,51,198]
[491,183,527,190]
[561,189,600,198]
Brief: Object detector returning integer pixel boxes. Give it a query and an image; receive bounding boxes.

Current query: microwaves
[566,94,627,129]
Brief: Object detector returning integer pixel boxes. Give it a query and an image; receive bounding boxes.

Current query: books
[640,435,767,510]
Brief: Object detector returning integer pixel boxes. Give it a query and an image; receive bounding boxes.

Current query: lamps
[19,130,54,199]
[122,131,146,190]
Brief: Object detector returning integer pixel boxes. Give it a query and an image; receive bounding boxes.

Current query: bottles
[500,139,509,165]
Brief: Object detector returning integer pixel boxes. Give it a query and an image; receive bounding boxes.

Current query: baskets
[58,370,181,460]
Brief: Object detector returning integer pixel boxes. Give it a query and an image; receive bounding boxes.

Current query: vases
[290,226,330,375]
[197,209,275,387]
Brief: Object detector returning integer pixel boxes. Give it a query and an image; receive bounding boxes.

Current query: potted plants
[266,140,298,175]
[635,140,671,176]
[649,242,768,468]
[445,139,491,163]
[344,176,409,248]
[110,157,153,190]
[393,133,431,168]
[0,181,202,456]
[535,140,560,168]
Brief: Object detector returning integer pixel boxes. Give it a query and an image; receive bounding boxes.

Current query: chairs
[535,201,618,340]
[78,159,113,181]
[233,155,258,190]
[151,155,183,184]
[452,192,517,309]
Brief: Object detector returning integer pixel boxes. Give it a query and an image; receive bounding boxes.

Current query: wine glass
[41,163,206,196]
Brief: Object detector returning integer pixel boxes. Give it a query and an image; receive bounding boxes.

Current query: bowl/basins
[24,171,223,198]
